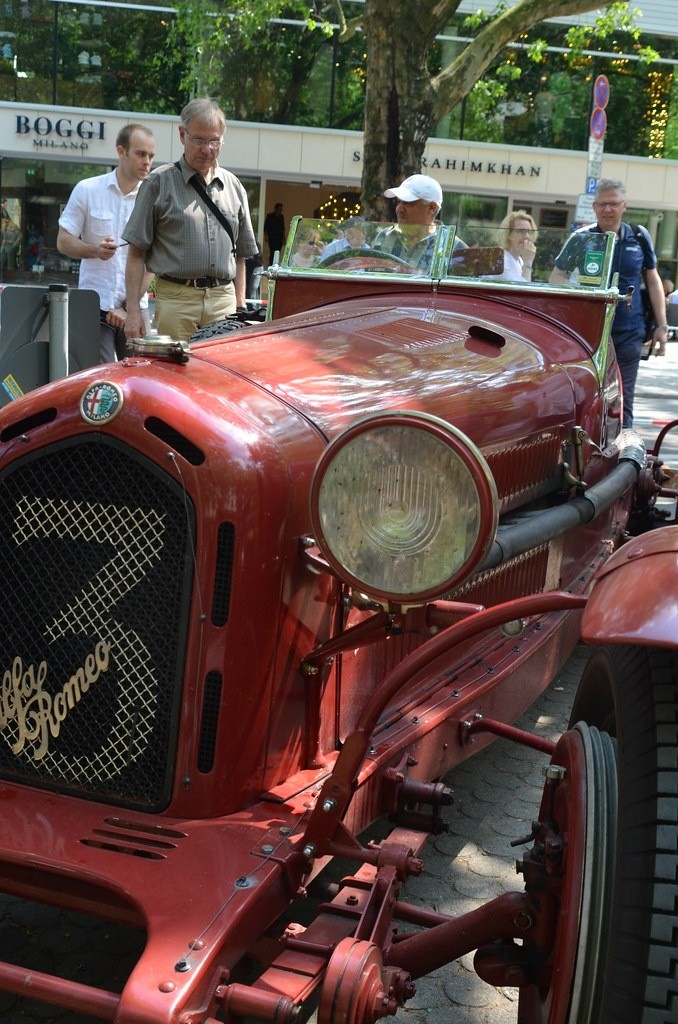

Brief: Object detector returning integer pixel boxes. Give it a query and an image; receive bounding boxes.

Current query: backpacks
[629,221,657,361]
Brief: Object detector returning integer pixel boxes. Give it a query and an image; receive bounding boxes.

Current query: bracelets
[521,264,533,270]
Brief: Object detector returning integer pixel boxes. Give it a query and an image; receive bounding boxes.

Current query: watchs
[656,323,670,332]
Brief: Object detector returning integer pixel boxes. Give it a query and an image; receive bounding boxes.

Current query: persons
[266,202,286,268]
[477,210,538,282]
[288,226,325,270]
[121,99,259,344]
[551,176,670,430]
[1,199,11,221]
[56,124,155,363]
[1,218,22,272]
[371,174,469,275]
[321,216,370,268]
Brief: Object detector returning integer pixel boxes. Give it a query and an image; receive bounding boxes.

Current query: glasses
[185,130,223,150]
[512,229,535,235]
[306,240,318,245]
[594,201,625,207]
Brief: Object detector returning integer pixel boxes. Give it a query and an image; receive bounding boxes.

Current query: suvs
[0,213,678,1024]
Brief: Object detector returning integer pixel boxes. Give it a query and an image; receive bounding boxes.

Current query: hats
[383,173,444,210]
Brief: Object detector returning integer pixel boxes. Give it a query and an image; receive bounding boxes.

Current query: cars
[37,246,73,274]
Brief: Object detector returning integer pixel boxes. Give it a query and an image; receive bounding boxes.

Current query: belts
[155,273,232,288]
